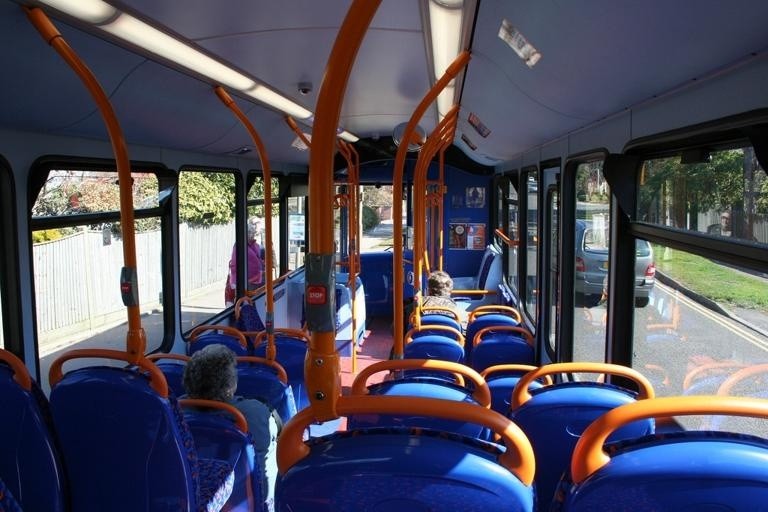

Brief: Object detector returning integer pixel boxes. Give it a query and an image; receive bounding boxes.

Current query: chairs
[481,364,552,419]
[408,307,463,340]
[595,365,672,398]
[186,325,248,367]
[645,324,689,395]
[235,356,297,424]
[138,354,194,397]
[467,305,521,359]
[48,349,235,512]
[561,397,768,512]
[510,362,654,512]
[252,328,323,425]
[706,364,768,440]
[347,359,492,442]
[683,363,752,397]
[470,326,535,391]
[276,396,537,511]
[405,326,464,379]
[177,399,268,512]
[234,296,267,355]
[0,349,72,512]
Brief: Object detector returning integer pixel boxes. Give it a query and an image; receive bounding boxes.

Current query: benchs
[452,244,503,312]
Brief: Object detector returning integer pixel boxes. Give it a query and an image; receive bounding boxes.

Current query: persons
[229,224,262,289]
[421,271,459,318]
[177,343,278,480]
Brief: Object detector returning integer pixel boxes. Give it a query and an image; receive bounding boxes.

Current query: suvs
[551,217,656,299]
